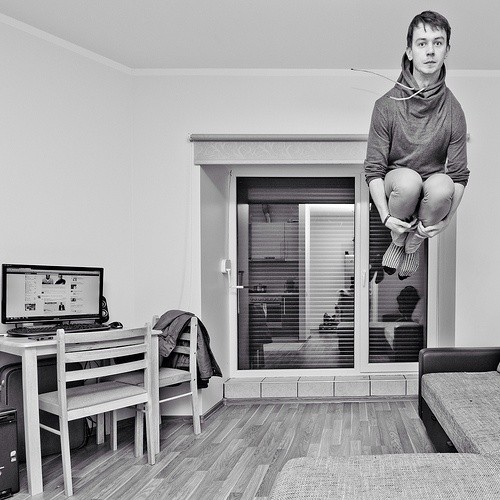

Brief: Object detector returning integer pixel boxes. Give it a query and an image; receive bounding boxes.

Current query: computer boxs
[0,404,20,500]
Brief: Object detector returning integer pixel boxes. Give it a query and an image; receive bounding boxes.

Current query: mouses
[110,321,123,328]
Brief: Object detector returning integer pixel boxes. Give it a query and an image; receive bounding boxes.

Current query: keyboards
[7,324,110,336]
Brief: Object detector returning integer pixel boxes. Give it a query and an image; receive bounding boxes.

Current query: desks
[0,325,165,495]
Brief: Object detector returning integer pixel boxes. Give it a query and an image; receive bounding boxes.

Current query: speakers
[95,296,109,323]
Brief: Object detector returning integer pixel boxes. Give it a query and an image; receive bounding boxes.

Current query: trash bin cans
[0,403,19,500]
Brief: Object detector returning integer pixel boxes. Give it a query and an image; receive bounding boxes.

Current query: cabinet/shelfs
[248,293,299,337]
[248,222,300,262]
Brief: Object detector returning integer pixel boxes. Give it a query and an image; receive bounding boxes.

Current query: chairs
[37,322,157,495]
[97,314,202,458]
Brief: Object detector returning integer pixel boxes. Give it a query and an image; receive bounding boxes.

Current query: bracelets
[384,214,391,225]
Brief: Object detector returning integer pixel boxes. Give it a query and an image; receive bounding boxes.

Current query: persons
[42,275,53,284]
[59,302,65,311]
[369,189,392,284]
[55,275,66,284]
[74,279,76,281]
[364,11,470,280]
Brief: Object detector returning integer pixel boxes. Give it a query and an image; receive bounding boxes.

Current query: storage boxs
[0,406,20,500]
[0,356,89,460]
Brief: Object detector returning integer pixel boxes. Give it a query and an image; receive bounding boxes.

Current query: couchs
[265,347,500,500]
[337,322,424,361]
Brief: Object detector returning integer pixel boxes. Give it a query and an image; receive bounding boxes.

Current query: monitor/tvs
[3,264,105,326]
